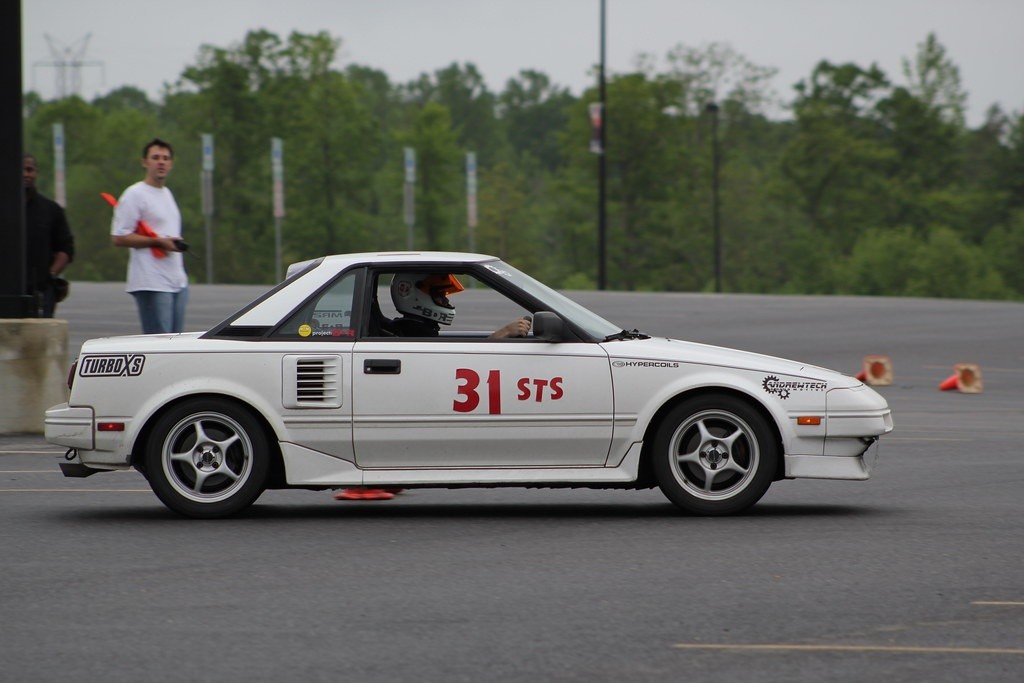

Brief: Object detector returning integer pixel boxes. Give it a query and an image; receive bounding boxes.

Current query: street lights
[702,98,722,291]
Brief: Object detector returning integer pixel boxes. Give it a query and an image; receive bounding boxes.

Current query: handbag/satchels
[51,272,69,302]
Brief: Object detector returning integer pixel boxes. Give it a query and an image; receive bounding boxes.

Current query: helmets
[390,269,456,326]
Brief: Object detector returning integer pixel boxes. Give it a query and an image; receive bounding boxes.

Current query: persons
[390,271,531,339]
[109,139,189,334]
[22,153,75,317]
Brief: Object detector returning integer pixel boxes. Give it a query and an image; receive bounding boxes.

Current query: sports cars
[40,249,895,521]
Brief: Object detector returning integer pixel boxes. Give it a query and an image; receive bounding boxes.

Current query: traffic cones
[336,487,394,501]
[852,354,896,387]
[938,364,982,396]
